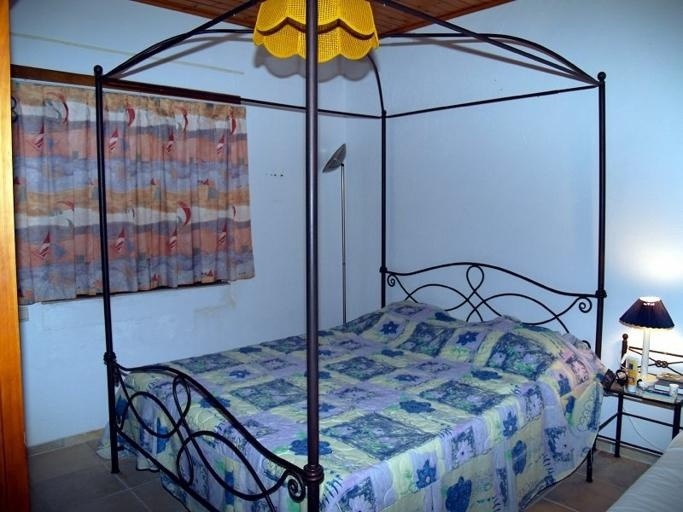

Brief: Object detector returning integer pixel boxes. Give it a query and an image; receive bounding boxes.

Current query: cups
[668,383,679,397]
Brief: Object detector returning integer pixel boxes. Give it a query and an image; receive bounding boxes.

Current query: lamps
[616,294,674,390]
[252,1,379,64]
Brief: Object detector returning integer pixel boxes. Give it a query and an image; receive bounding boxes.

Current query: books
[642,388,677,403]
[654,380,683,394]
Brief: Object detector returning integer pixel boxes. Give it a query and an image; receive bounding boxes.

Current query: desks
[608,335,683,461]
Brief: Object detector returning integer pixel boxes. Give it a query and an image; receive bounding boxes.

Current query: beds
[103,262,606,512]
